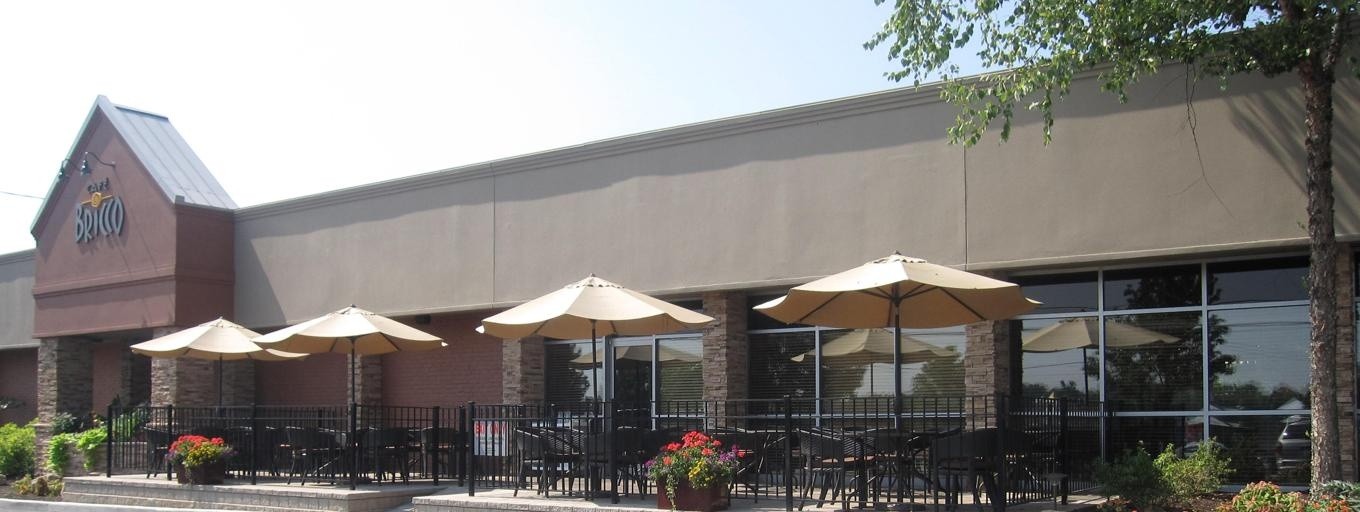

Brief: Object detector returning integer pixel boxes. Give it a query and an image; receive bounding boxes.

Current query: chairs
[142,400,1107,512]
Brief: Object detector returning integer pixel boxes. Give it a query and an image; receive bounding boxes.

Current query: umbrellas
[476,272,716,418]
[569,343,703,401]
[752,250,1045,399]
[250,305,447,403]
[131,316,308,405]
[1023,309,1181,406]
[789,325,957,396]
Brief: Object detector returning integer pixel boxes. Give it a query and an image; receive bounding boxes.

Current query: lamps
[57,158,81,181]
[79,151,116,177]
